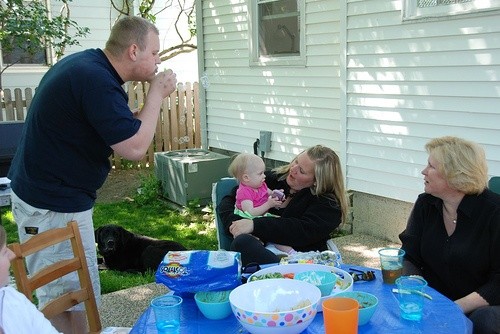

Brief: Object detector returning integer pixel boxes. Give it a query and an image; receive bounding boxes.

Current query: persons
[398,135,500,334]
[218,145,350,267]
[0,228,60,334]
[227,153,298,255]
[7,16,178,334]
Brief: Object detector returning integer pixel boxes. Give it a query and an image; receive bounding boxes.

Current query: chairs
[211,177,240,250]
[10,220,132,334]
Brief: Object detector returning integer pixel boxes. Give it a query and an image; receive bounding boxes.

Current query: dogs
[93,224,189,278]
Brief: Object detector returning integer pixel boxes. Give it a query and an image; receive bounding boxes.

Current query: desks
[130,264,474,334]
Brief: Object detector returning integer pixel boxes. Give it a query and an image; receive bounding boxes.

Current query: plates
[318,260,341,268]
[247,264,353,312]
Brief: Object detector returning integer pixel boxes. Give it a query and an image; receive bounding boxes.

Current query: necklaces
[443,208,457,224]
[288,192,297,197]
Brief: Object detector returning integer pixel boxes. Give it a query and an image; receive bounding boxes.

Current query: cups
[151,295,183,334]
[378,248,405,284]
[321,297,359,334]
[394,276,427,321]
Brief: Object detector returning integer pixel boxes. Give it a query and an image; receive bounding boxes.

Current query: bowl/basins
[229,278,321,334]
[333,291,378,326]
[194,290,232,320]
[293,270,336,296]
